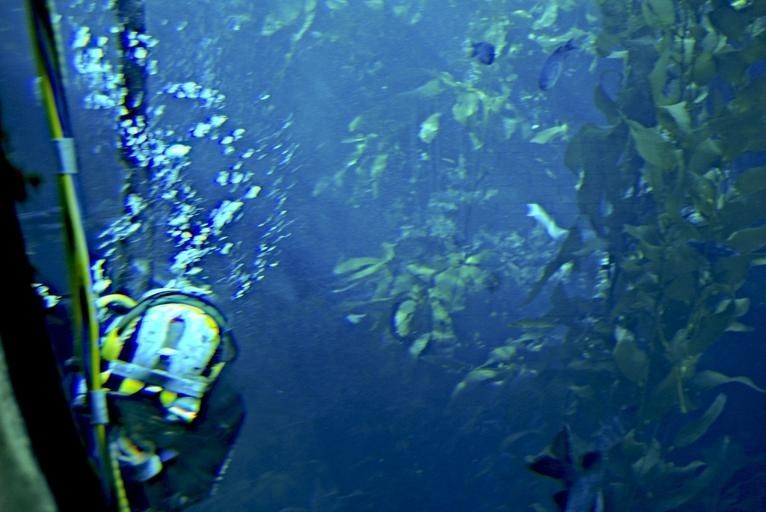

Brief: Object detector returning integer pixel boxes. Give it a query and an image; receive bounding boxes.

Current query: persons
[64,280,248,511]
